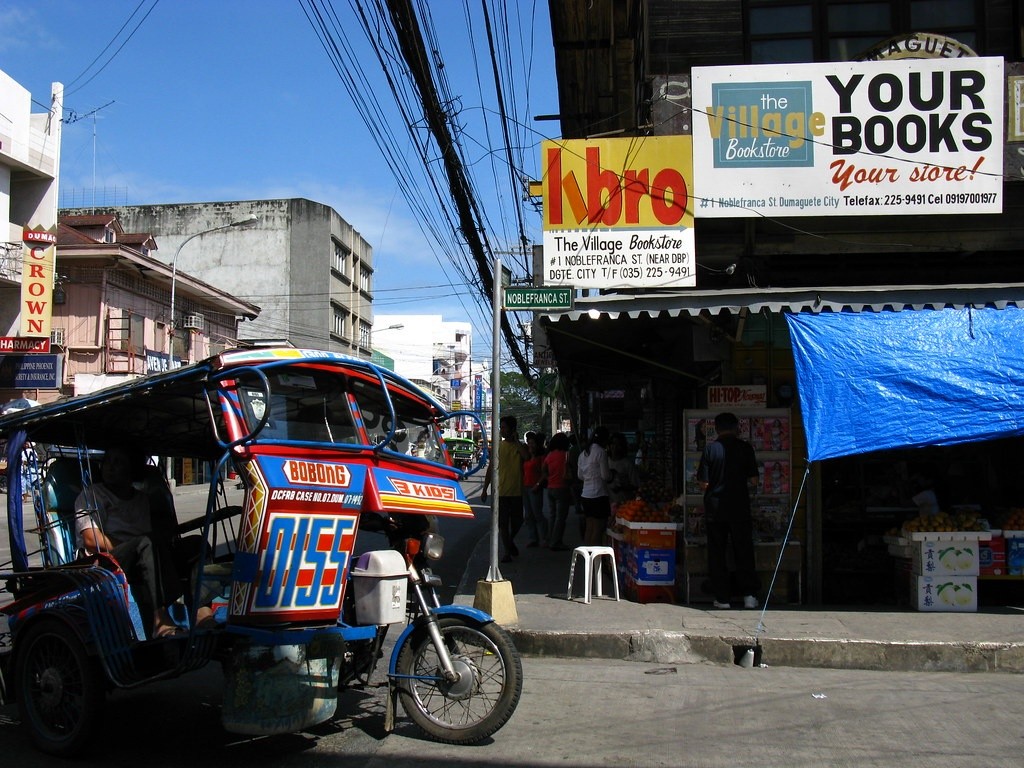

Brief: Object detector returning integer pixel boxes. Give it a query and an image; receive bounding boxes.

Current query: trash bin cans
[352,550,409,624]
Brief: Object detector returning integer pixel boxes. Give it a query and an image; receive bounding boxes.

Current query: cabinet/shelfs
[683,408,802,607]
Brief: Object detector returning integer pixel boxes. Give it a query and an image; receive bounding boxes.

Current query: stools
[567,546,620,604]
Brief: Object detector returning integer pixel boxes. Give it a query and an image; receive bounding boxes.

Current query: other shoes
[713,599,730,610]
[595,566,609,574]
[743,596,761,610]
[500,542,519,564]
[526,534,560,548]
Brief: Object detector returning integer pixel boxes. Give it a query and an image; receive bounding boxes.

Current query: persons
[686,418,786,545]
[21,439,39,503]
[480,451,487,466]
[74,437,224,642]
[522,427,682,564]
[480,416,532,563]
[697,412,767,610]
[461,460,469,479]
[405,431,439,463]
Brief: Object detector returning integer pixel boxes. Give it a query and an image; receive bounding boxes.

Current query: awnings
[534,284,1024,323]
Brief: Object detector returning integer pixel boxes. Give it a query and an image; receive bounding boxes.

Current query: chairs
[44,457,209,602]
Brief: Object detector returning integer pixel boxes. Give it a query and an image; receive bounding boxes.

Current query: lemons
[942,551,973,570]
[939,585,972,606]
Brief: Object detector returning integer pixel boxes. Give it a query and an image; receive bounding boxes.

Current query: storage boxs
[909,536,1024,611]
[606,525,675,604]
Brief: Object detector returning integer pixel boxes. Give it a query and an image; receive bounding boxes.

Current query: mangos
[890,511,983,535]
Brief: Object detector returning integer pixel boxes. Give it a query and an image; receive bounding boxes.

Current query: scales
[949,505,981,517]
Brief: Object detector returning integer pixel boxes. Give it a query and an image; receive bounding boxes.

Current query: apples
[1000,507,1024,530]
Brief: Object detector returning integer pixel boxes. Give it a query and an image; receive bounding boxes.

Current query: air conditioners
[184,316,204,330]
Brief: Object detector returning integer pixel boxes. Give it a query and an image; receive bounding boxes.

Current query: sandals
[151,623,182,637]
[196,616,219,627]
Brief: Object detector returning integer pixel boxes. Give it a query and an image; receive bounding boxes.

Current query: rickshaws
[0,336,524,750]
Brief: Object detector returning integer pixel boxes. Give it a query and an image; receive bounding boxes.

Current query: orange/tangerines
[616,500,671,522]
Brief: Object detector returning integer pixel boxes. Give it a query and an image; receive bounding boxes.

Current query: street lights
[166,214,260,372]
[356,323,405,359]
